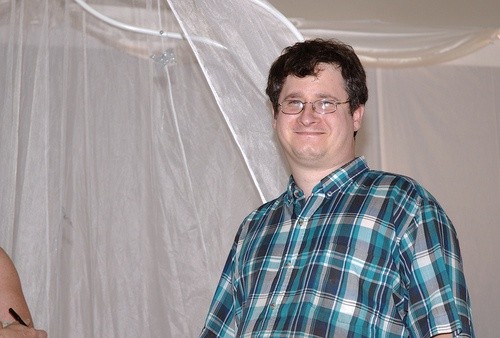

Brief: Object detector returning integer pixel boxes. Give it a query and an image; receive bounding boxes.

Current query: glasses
[278,99,350,114]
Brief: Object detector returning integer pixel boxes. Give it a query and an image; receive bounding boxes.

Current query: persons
[0,247,48,338]
[200,38,476,338]
